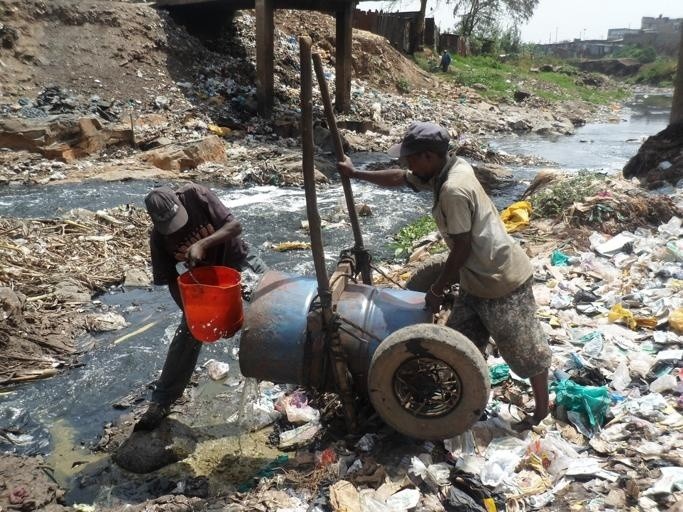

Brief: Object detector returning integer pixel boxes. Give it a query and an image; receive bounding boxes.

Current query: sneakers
[139,403,167,429]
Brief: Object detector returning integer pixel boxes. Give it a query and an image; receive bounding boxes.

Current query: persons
[333,119,554,432]
[438,49,451,72]
[131,180,273,431]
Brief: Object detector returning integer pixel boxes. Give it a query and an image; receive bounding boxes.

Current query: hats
[387,123,450,159]
[145,188,188,235]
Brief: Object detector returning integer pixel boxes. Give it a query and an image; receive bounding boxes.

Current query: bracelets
[429,283,444,298]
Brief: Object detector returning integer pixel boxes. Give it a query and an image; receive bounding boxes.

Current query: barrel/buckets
[176,262,245,344]
[238,269,434,397]
